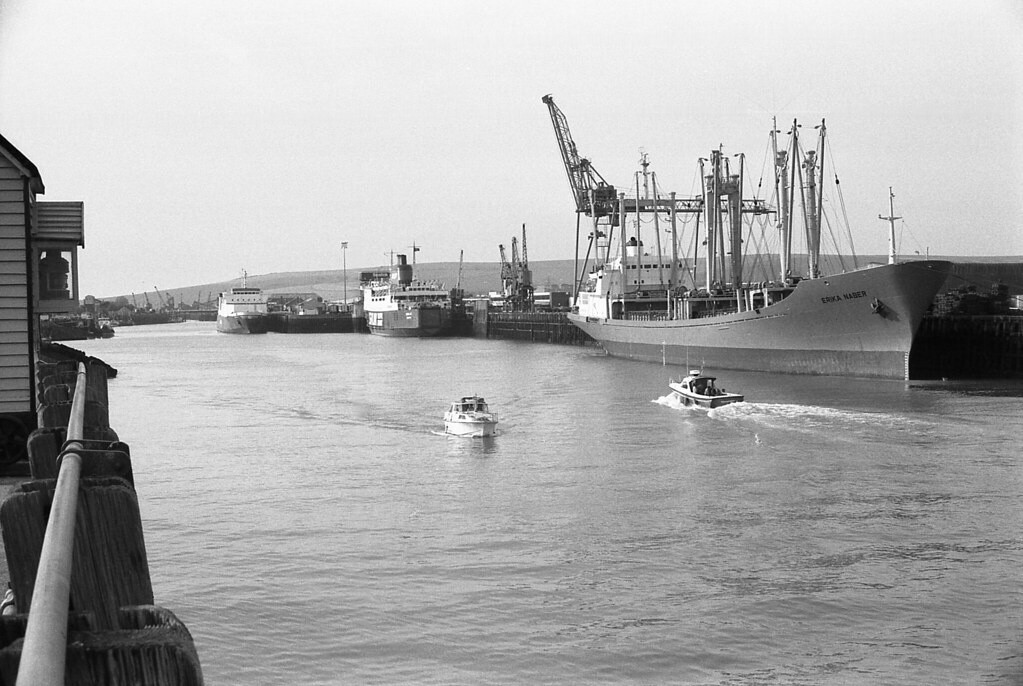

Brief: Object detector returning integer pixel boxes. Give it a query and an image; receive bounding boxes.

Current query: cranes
[498,222,537,313]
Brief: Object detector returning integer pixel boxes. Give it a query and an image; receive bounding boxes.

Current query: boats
[668,344,745,408]
[357,252,467,338]
[443,393,499,437]
[216,270,270,334]
[565,110,1023,382]
